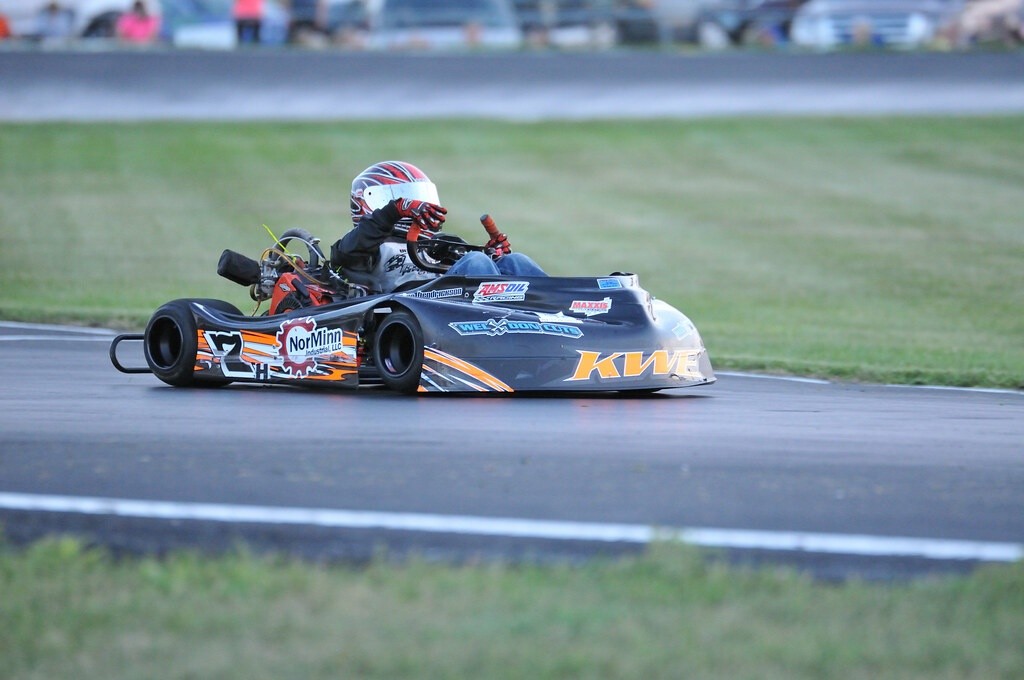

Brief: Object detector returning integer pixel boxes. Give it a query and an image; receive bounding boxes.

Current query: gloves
[484,233,512,259]
[396,199,447,229]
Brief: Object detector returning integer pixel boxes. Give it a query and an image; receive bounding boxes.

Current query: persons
[115,0,162,40]
[326,158,512,296]
[229,0,265,43]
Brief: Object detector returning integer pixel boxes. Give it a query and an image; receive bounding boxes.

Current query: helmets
[351,160,442,240]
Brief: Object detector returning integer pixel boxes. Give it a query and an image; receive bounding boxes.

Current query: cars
[790,0,964,48]
[326,0,619,51]
[0,0,162,39]
